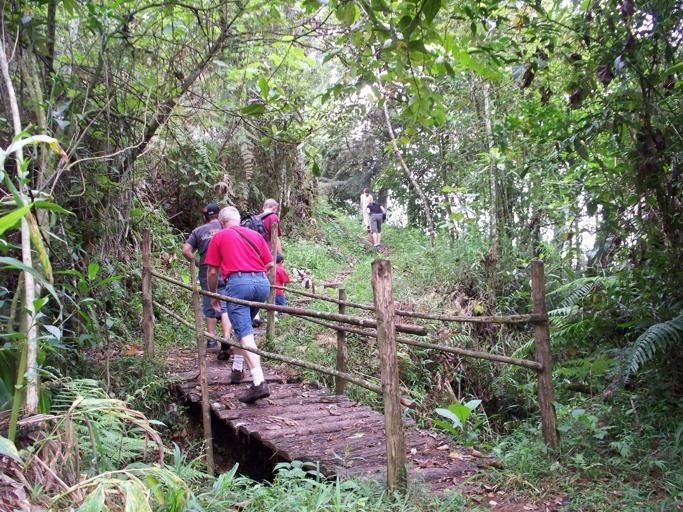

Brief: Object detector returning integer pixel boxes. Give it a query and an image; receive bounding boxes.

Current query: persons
[274,254,297,323]
[360,188,374,233]
[367,202,386,246]
[182,203,232,360]
[252,198,282,326]
[204,206,275,403]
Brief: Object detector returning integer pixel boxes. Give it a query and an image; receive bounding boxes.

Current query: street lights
[239,211,263,234]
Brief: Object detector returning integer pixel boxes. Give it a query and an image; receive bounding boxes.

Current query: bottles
[201,204,219,217]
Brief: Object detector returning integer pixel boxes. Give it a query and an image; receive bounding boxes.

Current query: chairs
[373,243,380,247]
[229,367,245,380]
[252,317,263,327]
[216,335,230,361]
[238,381,269,402]
[206,338,218,352]
